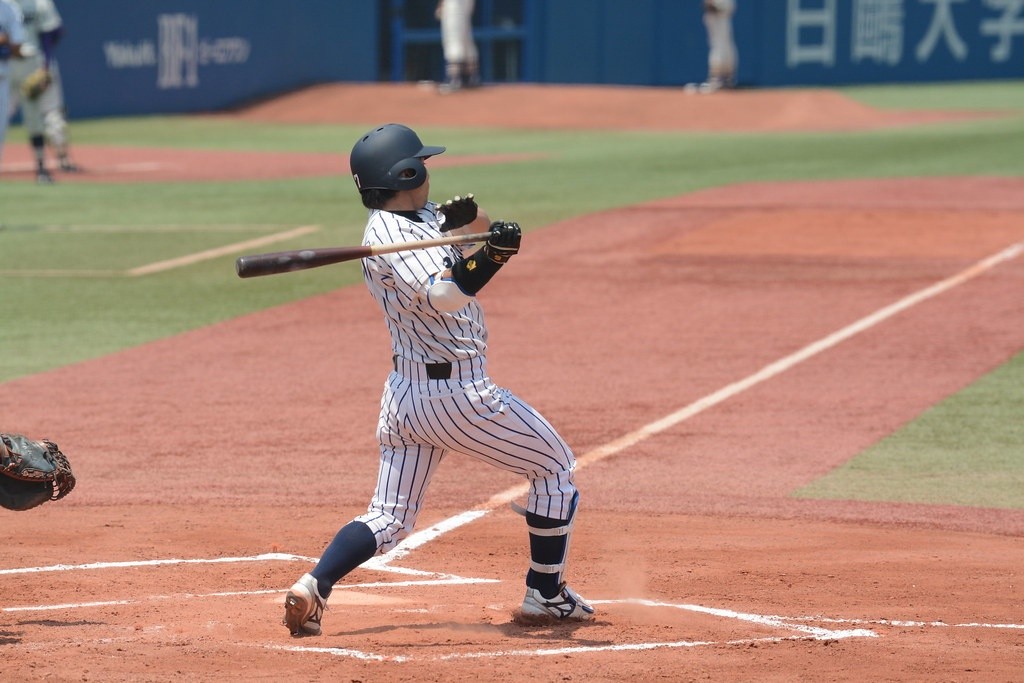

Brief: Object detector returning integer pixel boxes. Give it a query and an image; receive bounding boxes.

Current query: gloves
[486,220,521,264]
[435,193,478,232]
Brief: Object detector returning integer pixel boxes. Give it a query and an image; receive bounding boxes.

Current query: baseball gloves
[0,431,77,511]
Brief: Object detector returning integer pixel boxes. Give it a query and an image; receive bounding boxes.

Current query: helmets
[351,123,446,191]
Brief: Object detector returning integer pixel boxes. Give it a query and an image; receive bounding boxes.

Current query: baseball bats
[235,232,497,279]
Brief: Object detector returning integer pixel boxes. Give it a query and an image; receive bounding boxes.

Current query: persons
[701,0,739,90]
[0,0,84,184]
[434,0,481,89]
[282,123,596,635]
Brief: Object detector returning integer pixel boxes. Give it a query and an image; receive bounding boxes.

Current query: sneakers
[285,573,331,637]
[520,581,594,622]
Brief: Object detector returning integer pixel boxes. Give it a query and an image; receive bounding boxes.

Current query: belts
[393,356,452,379]
